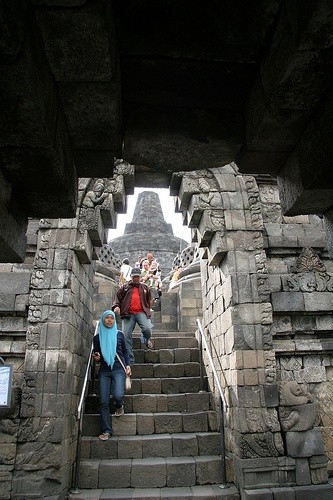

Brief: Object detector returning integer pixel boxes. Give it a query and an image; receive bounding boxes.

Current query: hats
[123,258,129,265]
[151,288,159,298]
[130,268,141,276]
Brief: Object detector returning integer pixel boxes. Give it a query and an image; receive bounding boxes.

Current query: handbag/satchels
[125,368,132,391]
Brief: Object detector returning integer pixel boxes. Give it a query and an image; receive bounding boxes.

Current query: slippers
[99,433,109,440]
[114,405,124,416]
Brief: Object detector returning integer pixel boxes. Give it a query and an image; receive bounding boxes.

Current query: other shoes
[147,340,153,348]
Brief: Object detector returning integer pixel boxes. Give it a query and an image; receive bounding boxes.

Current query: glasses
[144,265,147,266]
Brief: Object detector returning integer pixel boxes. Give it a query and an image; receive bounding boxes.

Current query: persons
[118,253,162,312]
[110,269,154,363]
[91,310,131,440]
